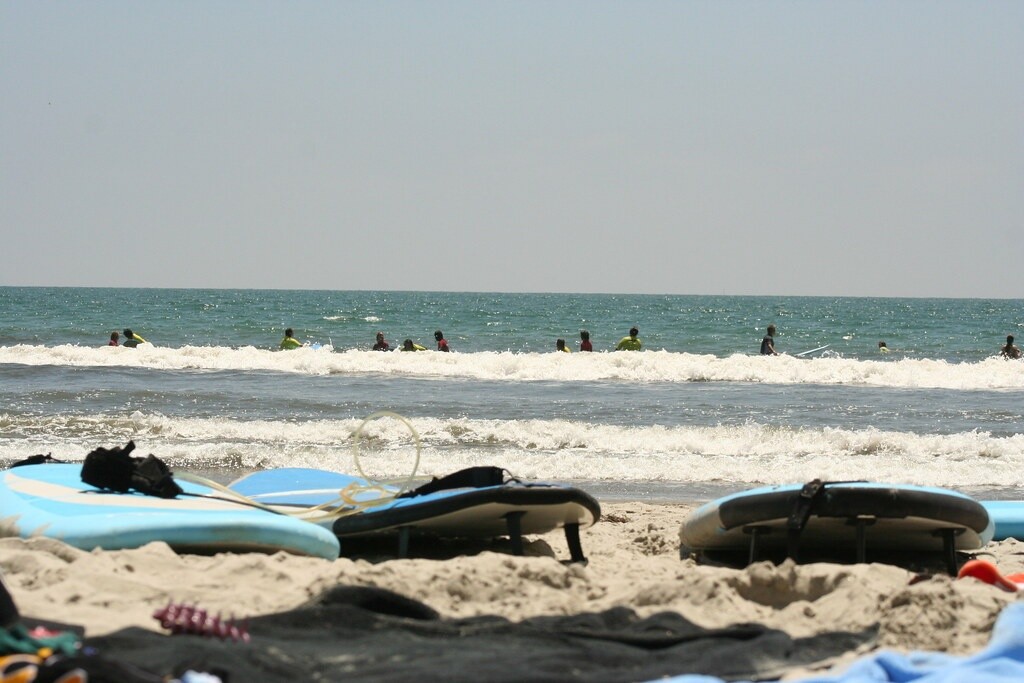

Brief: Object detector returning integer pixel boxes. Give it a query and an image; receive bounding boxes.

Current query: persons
[123,328,146,347]
[878,341,891,355]
[280,328,303,349]
[1002,333,1019,359]
[556,339,571,353]
[373,332,389,351]
[402,339,419,351]
[108,332,120,346]
[580,330,592,351]
[615,328,641,352]
[761,324,777,356]
[434,330,449,351]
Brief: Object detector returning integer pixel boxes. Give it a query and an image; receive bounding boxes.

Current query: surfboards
[976,498,1024,541]
[678,477,997,576]
[0,459,344,562]
[797,343,832,355]
[221,465,606,567]
